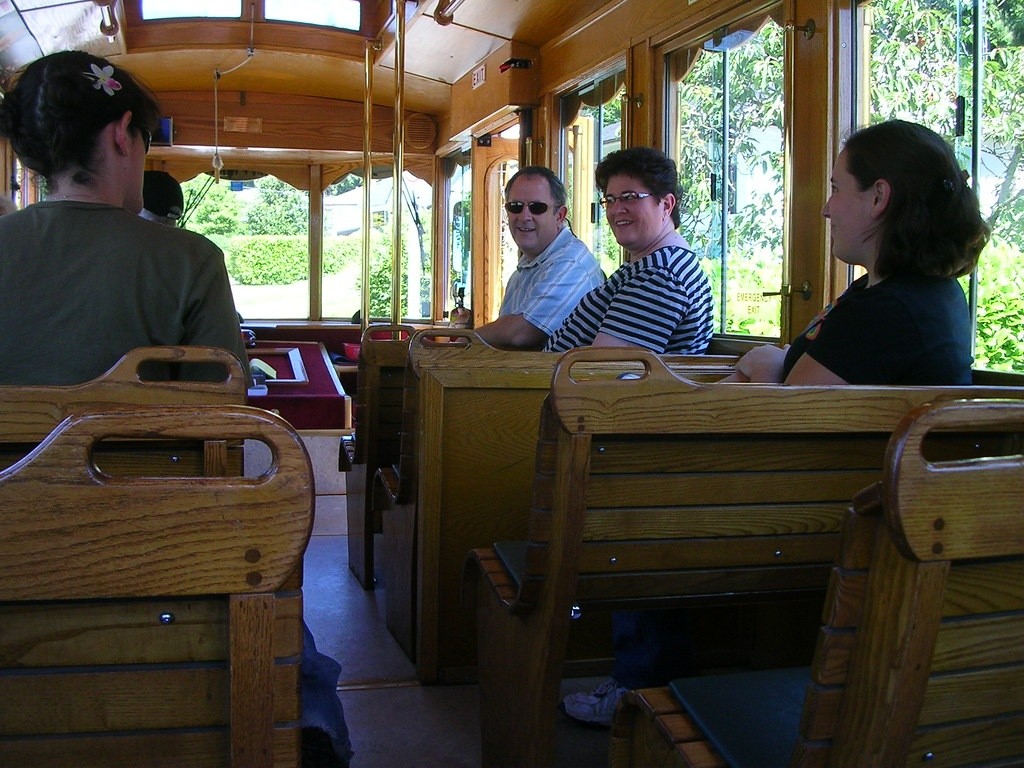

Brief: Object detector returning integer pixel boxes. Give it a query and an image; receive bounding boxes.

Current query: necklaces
[54,195,92,199]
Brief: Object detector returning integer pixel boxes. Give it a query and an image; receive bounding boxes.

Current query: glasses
[505,201,558,215]
[600,191,657,211]
[138,129,152,155]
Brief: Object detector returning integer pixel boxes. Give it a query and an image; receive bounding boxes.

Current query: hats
[143,170,184,220]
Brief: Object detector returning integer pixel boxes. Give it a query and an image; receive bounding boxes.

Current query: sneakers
[559,677,630,729]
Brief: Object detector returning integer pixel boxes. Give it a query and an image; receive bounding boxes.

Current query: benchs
[0,340,315,768]
[371,328,741,688]
[465,344,1024,768]
[338,325,543,592]
[618,382,1024,768]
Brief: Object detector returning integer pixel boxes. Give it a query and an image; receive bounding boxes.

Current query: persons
[539,145,712,355]
[564,120,993,726]
[457,166,604,351]
[0,53,354,767]
